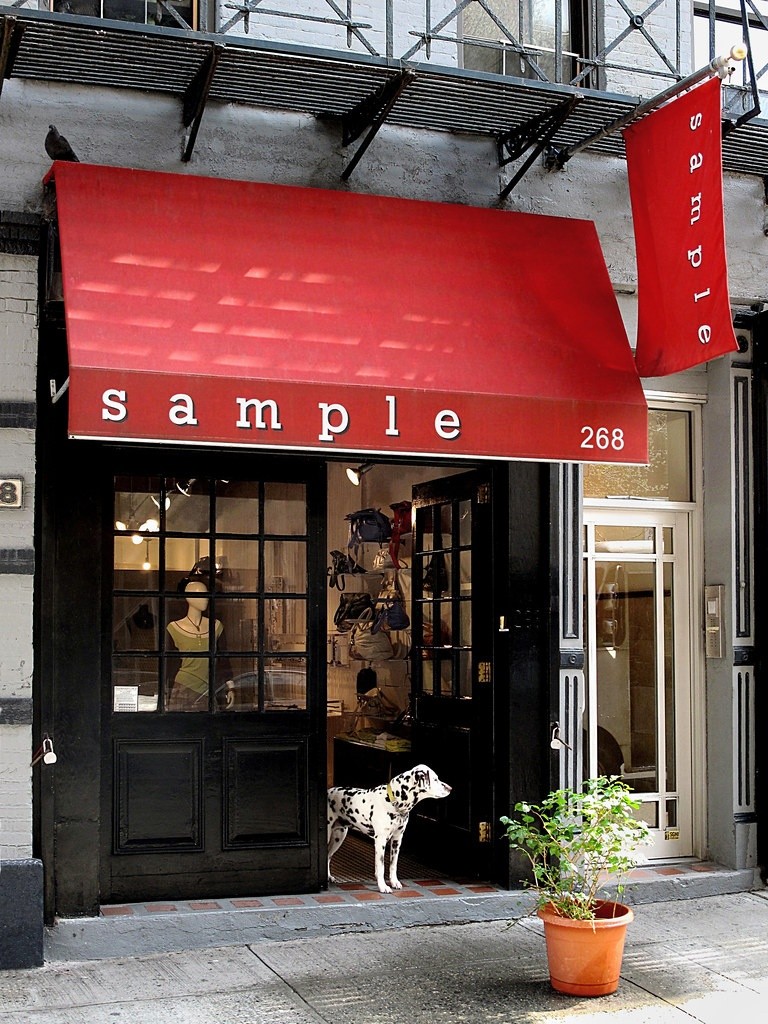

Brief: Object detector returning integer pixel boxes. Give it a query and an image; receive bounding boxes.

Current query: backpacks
[357,667,377,694]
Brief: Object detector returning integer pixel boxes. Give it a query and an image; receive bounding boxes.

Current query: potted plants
[500,773,654,995]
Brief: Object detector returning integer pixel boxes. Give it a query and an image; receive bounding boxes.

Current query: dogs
[326,764,452,894]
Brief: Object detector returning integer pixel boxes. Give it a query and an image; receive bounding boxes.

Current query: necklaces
[186,615,203,631]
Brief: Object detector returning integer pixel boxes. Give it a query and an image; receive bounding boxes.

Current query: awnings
[43,160,649,466]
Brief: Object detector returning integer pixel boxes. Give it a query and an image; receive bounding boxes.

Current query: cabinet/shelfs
[344,532,416,663]
[334,734,409,846]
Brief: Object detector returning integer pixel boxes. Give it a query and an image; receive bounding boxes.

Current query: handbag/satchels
[359,687,402,716]
[327,495,451,665]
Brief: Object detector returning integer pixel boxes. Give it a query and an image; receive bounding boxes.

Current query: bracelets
[228,687,236,693]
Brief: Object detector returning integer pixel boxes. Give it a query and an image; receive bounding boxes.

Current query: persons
[166,582,236,714]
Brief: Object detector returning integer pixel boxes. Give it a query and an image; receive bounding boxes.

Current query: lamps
[115,474,197,545]
[345,463,376,486]
